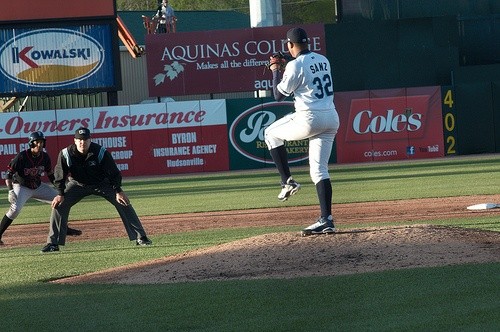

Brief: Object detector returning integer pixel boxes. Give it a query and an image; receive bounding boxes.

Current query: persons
[41,127,153,253]
[156,0,174,33]
[0,131,82,244]
[263,28,340,233]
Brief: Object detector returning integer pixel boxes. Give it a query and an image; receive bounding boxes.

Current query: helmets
[29,131,46,149]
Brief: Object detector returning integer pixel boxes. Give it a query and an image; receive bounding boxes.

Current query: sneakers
[301,218,335,234]
[135,237,152,245]
[40,243,59,253]
[277,176,300,203]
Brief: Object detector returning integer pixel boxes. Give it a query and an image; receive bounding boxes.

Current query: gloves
[8,190,17,205]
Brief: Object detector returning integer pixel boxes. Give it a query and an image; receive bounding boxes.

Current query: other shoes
[0,239,4,245]
[66,227,82,236]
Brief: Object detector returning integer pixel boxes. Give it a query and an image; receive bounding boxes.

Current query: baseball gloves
[266,51,296,73]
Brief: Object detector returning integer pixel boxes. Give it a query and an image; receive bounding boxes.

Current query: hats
[74,127,90,139]
[283,27,307,45]
